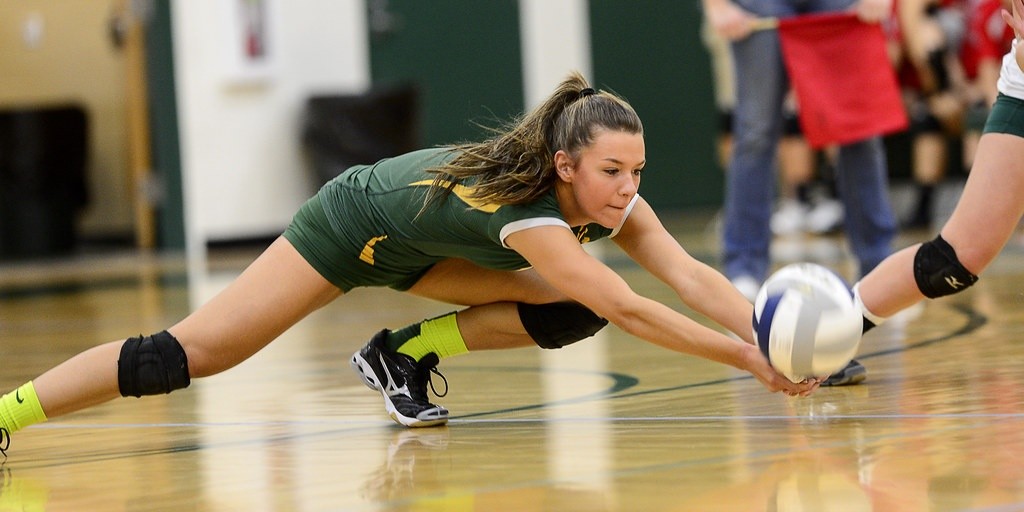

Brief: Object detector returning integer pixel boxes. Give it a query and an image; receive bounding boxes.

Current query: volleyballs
[749,258,867,385]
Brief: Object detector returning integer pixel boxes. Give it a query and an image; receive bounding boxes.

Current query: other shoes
[900,207,934,229]
[731,275,760,304]
[820,359,866,386]
[768,183,846,234]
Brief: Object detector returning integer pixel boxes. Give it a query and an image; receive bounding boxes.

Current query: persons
[882,3,1024,231]
[0,73,822,466]
[819,2,1023,385]
[714,0,925,324]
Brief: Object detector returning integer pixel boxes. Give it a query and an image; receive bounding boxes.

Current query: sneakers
[351,328,449,427]
